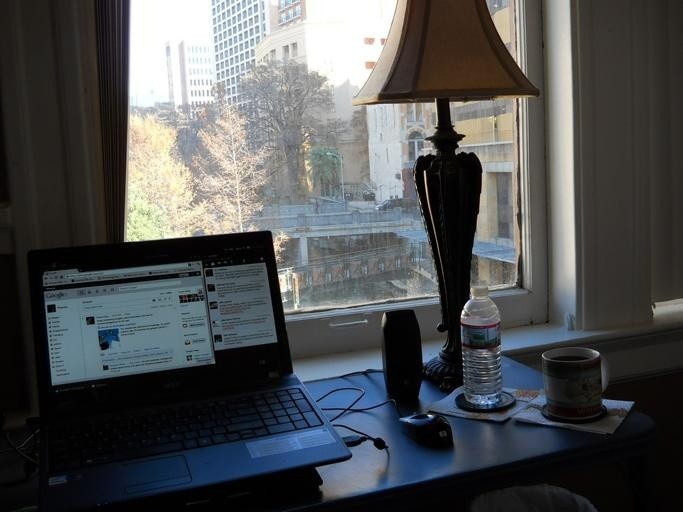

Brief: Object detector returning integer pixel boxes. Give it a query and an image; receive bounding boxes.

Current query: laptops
[24,229,354,511]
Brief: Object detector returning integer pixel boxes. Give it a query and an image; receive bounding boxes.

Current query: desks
[0,352,657,512]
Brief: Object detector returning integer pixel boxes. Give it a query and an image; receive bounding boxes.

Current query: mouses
[398,411,457,452]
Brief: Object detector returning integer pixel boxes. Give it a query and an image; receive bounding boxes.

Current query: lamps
[351,1,540,393]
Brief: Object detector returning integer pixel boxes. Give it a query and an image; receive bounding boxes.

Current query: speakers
[382,306,424,403]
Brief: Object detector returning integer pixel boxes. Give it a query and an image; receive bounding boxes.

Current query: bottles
[460,286,503,406]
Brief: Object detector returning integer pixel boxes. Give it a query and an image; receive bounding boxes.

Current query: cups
[541,347,603,416]
